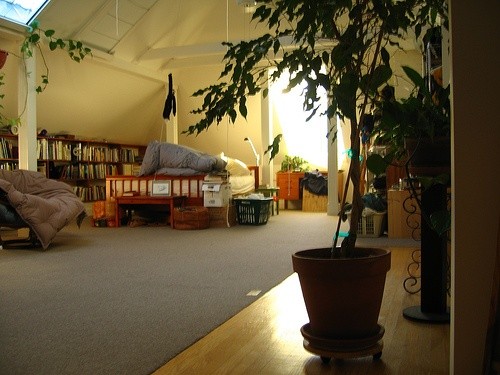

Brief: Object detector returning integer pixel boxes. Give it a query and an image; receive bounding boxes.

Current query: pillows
[158,168,200,176]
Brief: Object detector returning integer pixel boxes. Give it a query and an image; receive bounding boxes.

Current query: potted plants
[280,155,310,173]
[181,0,451,338]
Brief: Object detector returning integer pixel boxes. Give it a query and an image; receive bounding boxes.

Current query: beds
[107,166,259,228]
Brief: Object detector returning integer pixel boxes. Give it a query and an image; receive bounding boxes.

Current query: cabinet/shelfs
[0,134,148,202]
[278,171,343,209]
[387,189,424,238]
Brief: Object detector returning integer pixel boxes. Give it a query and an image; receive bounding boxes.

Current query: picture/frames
[152,180,172,196]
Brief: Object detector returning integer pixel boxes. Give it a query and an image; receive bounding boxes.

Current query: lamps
[244,137,260,166]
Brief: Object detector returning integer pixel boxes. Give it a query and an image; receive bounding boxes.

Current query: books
[37,138,144,202]
[0,137,19,170]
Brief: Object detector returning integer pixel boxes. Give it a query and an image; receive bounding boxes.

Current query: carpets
[0,209,422,375]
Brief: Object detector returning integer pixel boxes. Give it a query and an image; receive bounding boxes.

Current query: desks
[114,194,186,229]
[257,189,280,216]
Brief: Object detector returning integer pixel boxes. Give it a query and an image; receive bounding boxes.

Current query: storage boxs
[174,206,209,230]
[207,206,237,228]
[234,199,273,225]
[303,183,328,212]
[202,184,232,207]
[344,206,387,238]
